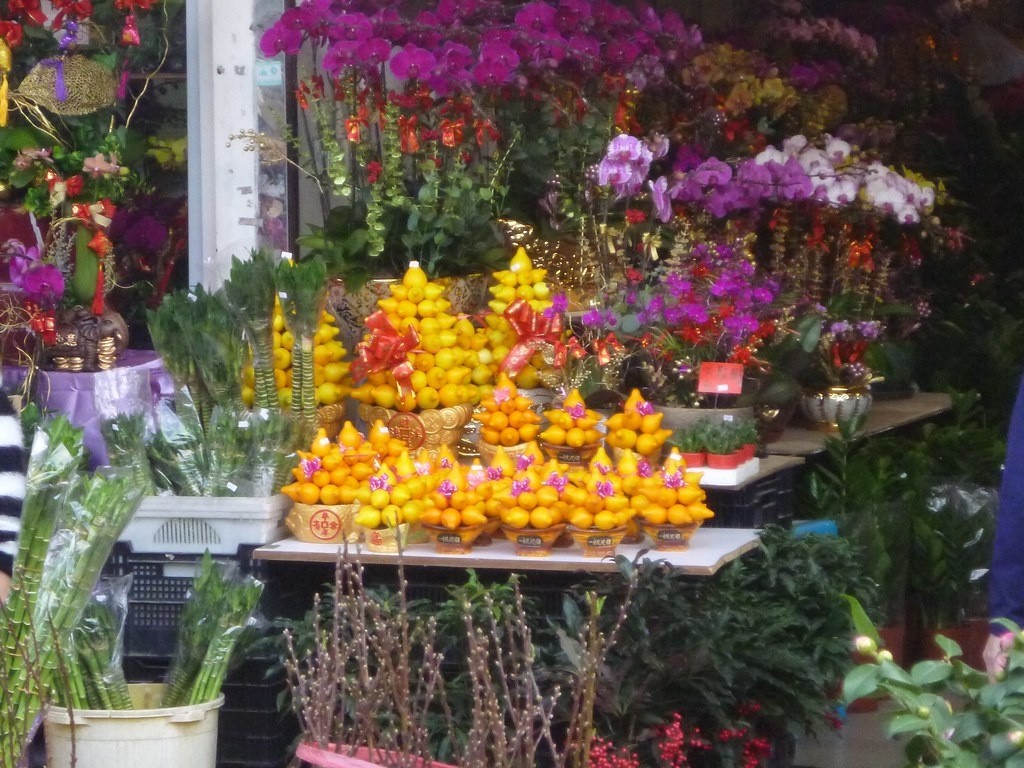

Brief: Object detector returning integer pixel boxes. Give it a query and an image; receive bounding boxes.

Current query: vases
[74,220,116,302]
[802,385,873,433]
[707,367,801,443]
[655,408,754,441]
[864,335,913,400]
[34,298,130,372]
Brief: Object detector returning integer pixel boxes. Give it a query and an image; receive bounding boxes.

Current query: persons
[984,387,1024,684]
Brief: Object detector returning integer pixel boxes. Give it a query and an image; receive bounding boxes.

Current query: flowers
[0,0,179,313]
[222,0,968,387]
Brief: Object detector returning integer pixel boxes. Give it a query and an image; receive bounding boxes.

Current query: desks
[253,524,770,577]
[684,454,804,489]
[762,393,954,456]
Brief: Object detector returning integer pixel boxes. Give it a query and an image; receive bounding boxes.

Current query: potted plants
[744,418,757,460]
[676,424,705,467]
[810,430,904,713]
[923,486,991,684]
[735,423,746,464]
[702,424,740,470]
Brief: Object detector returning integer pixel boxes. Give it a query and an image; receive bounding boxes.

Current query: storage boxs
[361,582,632,685]
[102,539,301,656]
[115,495,292,557]
[127,645,298,768]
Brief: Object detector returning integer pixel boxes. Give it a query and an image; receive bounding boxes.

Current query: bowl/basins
[541,443,602,471]
[421,516,698,558]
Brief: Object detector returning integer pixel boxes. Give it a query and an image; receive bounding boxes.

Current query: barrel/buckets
[42,683,225,768]
